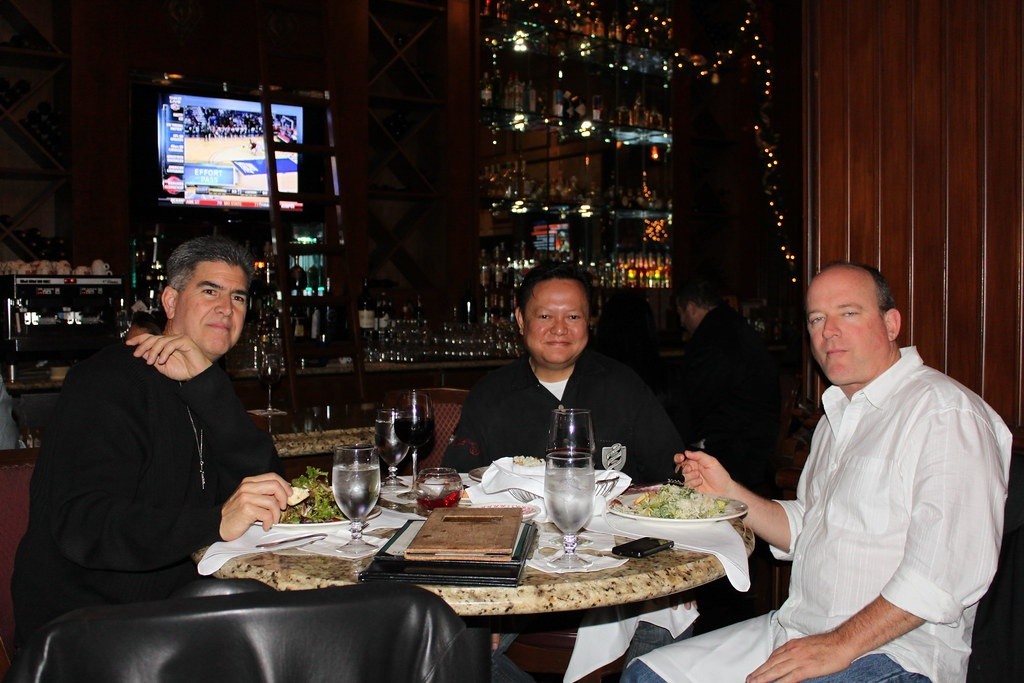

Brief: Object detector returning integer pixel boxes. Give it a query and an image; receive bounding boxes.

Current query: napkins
[197,456,789,683]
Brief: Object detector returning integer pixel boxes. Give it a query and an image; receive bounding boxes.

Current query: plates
[249,507,385,527]
[468,467,494,482]
[609,493,749,522]
[470,503,541,521]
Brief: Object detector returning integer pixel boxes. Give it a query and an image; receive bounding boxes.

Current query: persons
[11,234,283,656]
[441,264,703,683]
[123,311,161,342]
[620,261,1014,683]
[670,279,783,629]
[183,106,266,156]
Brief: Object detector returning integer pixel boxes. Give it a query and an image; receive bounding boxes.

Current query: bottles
[119,0,688,367]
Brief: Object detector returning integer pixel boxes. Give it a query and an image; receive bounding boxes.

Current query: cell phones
[611,537,674,558]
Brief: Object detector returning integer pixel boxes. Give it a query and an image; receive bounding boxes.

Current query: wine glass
[332,444,379,553]
[394,390,435,500]
[252,339,280,413]
[373,408,411,489]
[544,449,595,569]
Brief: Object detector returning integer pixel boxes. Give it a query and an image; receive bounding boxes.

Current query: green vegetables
[629,484,727,518]
[281,467,343,524]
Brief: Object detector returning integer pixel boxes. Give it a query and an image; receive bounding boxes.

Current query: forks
[666,437,708,485]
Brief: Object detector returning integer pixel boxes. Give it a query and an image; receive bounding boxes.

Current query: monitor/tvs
[127,75,327,225]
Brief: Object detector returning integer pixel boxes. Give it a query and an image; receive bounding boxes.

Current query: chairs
[1,448,45,681]
[400,386,472,470]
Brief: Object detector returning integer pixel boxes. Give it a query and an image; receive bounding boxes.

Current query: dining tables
[191,473,755,683]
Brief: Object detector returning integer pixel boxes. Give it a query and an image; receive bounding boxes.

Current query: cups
[547,407,595,469]
[411,467,462,513]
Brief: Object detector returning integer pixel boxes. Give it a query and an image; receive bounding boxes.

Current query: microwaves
[0,275,124,383]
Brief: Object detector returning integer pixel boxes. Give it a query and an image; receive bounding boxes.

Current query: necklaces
[177,379,207,490]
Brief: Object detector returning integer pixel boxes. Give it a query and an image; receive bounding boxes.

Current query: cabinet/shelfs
[1,0,71,267]
[469,15,674,376]
[365,1,451,307]
